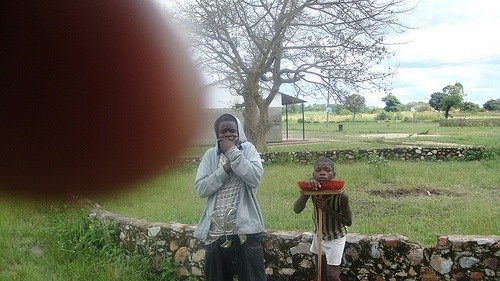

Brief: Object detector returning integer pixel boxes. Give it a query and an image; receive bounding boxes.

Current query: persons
[193,113,269,281]
[293,155,353,281]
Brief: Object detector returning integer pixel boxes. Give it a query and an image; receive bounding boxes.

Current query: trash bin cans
[339,124,344,132]
